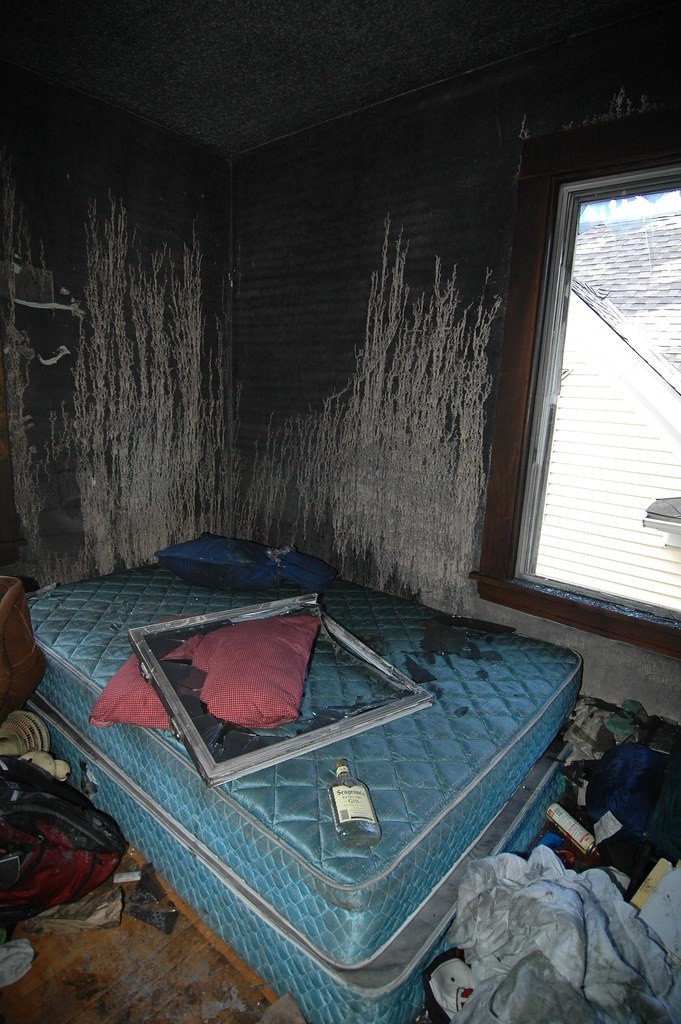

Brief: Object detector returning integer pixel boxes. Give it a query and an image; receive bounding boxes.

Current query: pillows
[153,531,285,596]
[84,613,321,730]
[270,550,339,589]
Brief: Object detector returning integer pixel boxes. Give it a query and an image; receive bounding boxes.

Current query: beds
[25,563,582,1024]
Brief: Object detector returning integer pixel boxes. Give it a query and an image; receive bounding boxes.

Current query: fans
[0,711,71,783]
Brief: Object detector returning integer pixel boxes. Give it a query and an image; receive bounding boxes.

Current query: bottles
[545,802,601,857]
[326,757,381,847]
